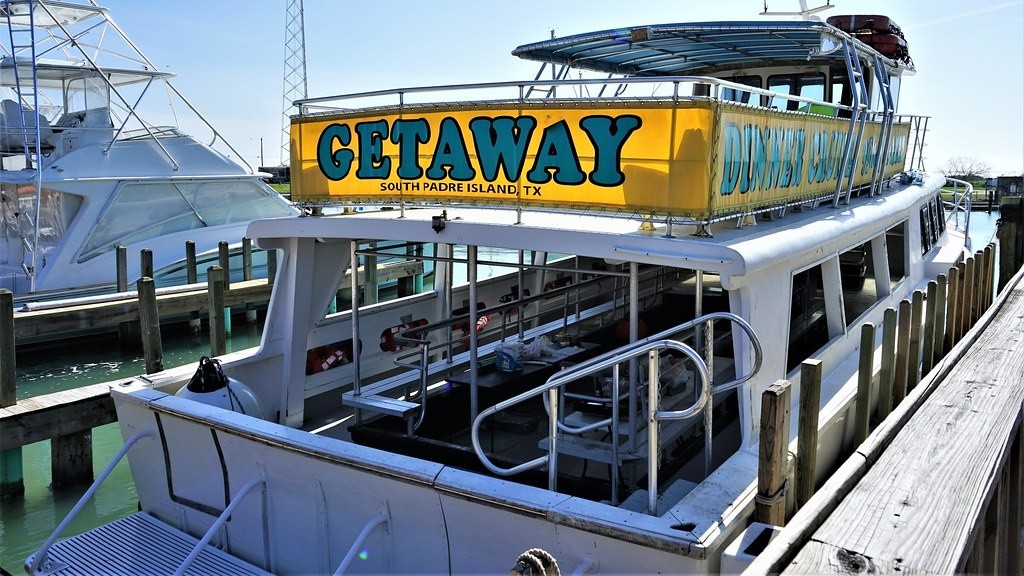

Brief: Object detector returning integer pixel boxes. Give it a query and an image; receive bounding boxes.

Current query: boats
[0,0,435,347]
[26,0,973,576]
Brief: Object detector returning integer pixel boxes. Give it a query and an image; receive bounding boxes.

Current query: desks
[540,358,734,484]
[448,342,601,455]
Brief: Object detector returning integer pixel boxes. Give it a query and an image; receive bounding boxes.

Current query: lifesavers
[465,315,489,345]
